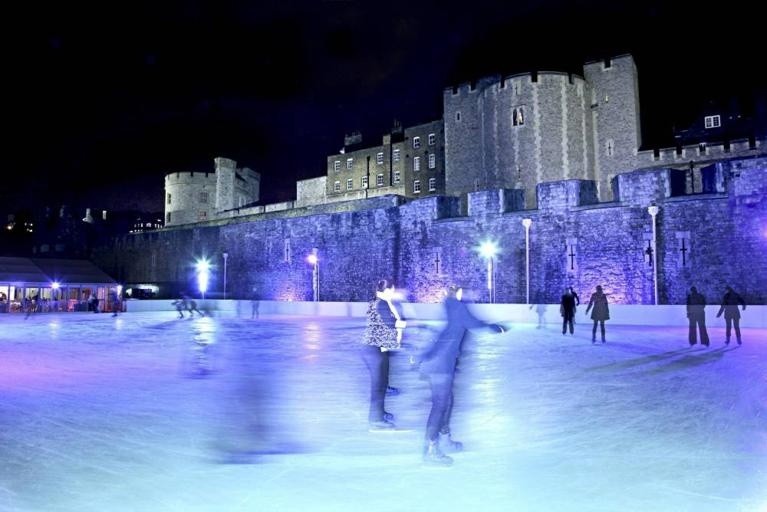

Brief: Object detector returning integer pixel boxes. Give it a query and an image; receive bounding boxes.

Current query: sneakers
[368,412,394,429]
[386,387,399,394]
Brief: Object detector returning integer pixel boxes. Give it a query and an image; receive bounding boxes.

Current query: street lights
[221,252,228,299]
[647,203,659,305]
[522,217,532,302]
[196,260,208,299]
[479,239,497,303]
[307,246,319,301]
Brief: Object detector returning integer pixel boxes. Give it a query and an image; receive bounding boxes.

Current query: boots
[422,440,454,464]
[437,431,462,454]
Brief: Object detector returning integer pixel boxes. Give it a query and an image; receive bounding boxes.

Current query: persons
[562,290,575,334]
[384,282,410,395]
[568,287,580,324]
[530,290,548,328]
[250,288,261,318]
[172,292,192,318]
[687,287,710,346]
[586,286,610,342]
[716,286,745,345]
[0,293,120,319]
[360,280,421,428]
[411,288,512,464]
[189,297,204,317]
